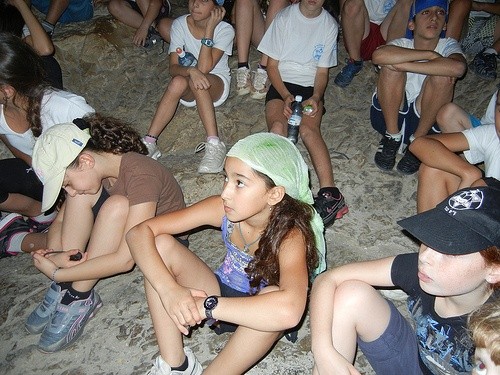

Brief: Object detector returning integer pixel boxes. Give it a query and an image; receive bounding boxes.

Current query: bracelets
[52,267,62,284]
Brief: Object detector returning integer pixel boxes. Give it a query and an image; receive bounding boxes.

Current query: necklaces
[238,221,262,254]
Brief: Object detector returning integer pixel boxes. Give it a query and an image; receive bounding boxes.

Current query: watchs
[203,295,221,319]
[201,38,215,47]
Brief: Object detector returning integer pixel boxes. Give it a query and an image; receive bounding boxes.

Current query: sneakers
[374,136,401,171]
[310,190,348,230]
[0,211,53,258]
[27,282,102,354]
[334,60,362,87]
[141,138,162,160]
[235,66,272,98]
[467,49,497,80]
[197,141,227,174]
[396,145,421,175]
[144,349,202,375]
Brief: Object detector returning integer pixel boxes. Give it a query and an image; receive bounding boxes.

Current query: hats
[397,186,499,255]
[31,122,91,214]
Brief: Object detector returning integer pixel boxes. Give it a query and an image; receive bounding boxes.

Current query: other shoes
[141,27,161,47]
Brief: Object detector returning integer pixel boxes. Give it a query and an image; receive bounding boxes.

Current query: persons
[222,0,299,99]
[125,132,328,375]
[435,87,500,163]
[333,0,415,88]
[369,0,467,175]
[106,0,171,51]
[256,0,349,230]
[308,186,500,375]
[445,0,500,82]
[407,87,500,214]
[466,298,500,375]
[23,0,95,39]
[139,0,236,174]
[158,17,176,44]
[24,112,191,355]
[0,30,96,261]
[0,0,55,56]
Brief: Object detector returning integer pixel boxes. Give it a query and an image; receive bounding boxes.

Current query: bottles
[287,95,304,145]
[177,47,198,72]
